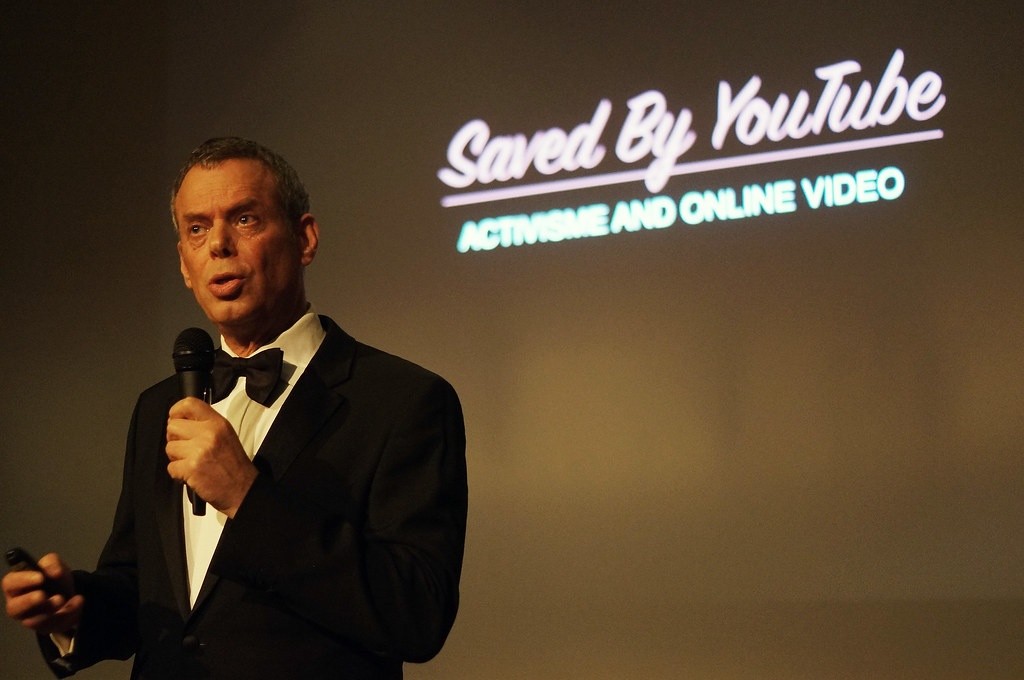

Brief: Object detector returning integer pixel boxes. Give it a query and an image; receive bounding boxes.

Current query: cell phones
[4,548,69,606]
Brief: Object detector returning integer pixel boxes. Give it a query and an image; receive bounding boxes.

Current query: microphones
[170,326,215,517]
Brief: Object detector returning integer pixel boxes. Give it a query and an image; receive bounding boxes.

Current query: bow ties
[204,347,285,408]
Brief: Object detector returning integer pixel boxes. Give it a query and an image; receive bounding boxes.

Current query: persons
[3,138,468,680]
